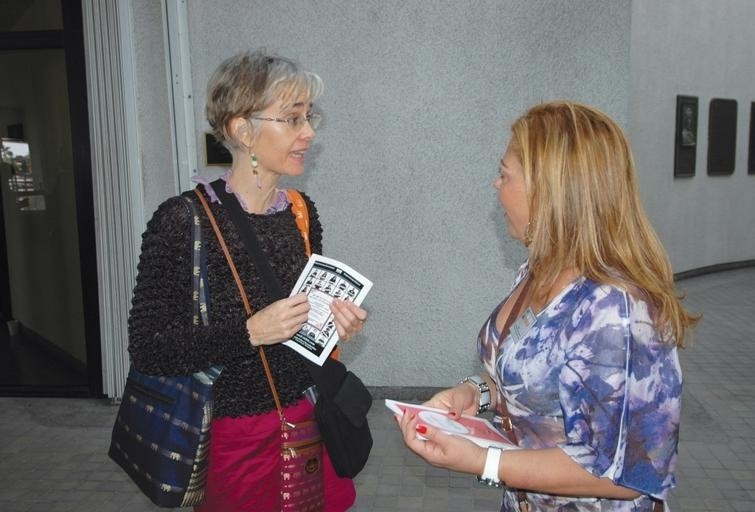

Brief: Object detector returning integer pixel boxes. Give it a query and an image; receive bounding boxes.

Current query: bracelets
[477,440,506,488]
[461,375,491,415]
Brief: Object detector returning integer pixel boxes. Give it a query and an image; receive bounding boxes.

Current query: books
[384,396,521,454]
[281,253,373,369]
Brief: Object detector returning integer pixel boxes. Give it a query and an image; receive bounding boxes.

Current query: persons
[127,52,367,512]
[392,103,703,512]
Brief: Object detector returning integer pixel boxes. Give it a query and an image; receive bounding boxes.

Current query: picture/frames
[707,97,737,179]
[674,93,699,181]
[746,100,753,177]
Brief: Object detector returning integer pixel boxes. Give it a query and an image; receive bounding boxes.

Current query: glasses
[253,114,321,131]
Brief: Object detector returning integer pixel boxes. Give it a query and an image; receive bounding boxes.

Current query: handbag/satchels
[280,422,325,511]
[109,365,223,508]
